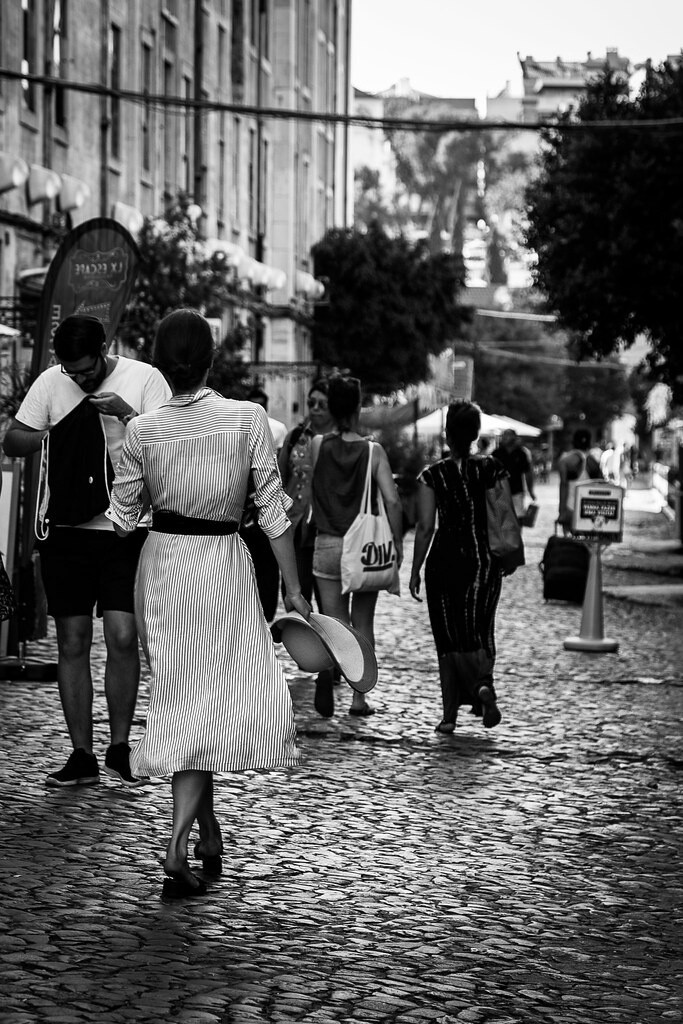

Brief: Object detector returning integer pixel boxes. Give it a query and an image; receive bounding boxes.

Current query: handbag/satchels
[33,396,115,541]
[522,505,538,527]
[342,510,401,598]
[486,471,525,577]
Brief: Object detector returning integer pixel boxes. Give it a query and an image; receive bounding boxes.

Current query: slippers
[194,841,222,877]
[314,671,334,717]
[162,872,206,899]
[349,703,375,716]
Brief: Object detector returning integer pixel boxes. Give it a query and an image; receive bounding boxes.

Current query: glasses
[61,355,98,377]
[307,399,327,411]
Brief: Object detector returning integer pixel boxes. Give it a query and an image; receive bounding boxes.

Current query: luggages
[539,520,590,607]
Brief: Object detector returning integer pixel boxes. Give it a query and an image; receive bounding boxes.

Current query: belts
[152,510,239,541]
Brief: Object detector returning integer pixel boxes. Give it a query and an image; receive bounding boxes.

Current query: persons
[101,302,317,901]
[0,314,671,790]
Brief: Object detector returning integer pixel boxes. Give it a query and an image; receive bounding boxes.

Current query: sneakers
[46,748,100,786]
[103,742,142,787]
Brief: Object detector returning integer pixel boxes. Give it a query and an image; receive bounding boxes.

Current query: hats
[269,611,378,694]
[309,379,330,397]
[54,315,106,361]
[497,429,521,444]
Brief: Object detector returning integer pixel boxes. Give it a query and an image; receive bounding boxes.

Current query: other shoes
[435,719,456,735]
[479,686,501,728]
[332,667,341,684]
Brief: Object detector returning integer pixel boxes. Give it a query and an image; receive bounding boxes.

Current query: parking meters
[564,479,625,654]
